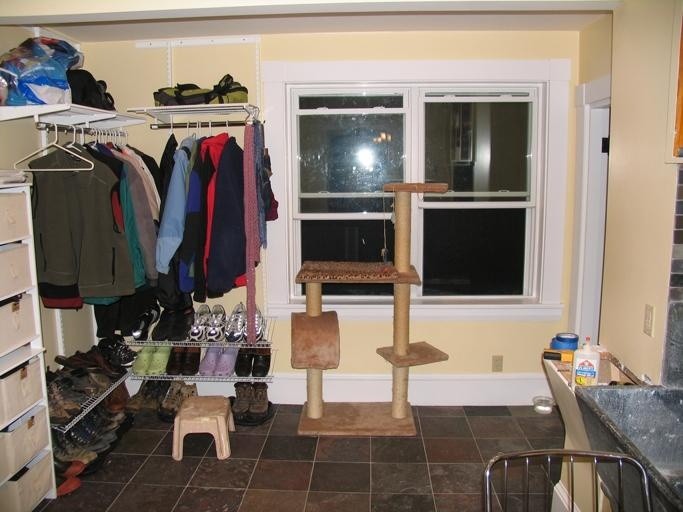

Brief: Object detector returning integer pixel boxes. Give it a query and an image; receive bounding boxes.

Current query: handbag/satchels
[153,72,248,107]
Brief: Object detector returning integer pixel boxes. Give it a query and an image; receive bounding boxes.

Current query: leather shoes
[45,334,138,497]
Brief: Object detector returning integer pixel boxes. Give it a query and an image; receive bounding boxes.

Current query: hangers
[167,120,230,139]
[13,122,128,173]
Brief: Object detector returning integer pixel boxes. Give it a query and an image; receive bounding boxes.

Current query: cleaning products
[571,337,600,392]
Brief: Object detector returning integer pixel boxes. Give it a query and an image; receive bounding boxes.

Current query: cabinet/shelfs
[127,314,277,384]
[0,181,57,512]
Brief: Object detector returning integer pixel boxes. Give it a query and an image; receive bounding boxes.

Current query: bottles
[552,333,600,392]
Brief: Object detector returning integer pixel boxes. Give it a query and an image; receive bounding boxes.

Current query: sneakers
[230,382,252,415]
[126,377,198,421]
[131,301,271,341]
[249,382,268,415]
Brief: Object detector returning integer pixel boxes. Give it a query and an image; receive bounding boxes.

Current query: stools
[170,395,236,461]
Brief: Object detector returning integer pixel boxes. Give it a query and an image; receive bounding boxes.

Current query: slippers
[131,343,275,379]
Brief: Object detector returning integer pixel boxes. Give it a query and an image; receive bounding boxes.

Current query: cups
[534,396,555,415]
